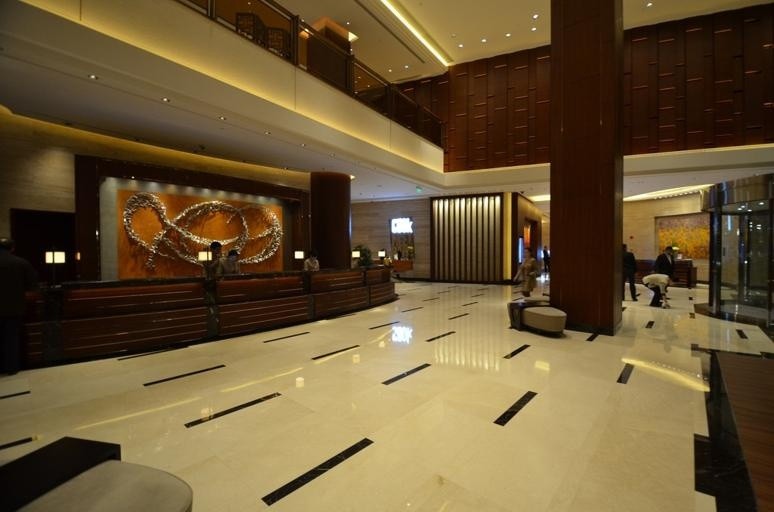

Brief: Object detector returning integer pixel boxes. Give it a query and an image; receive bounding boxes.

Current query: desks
[713,351,774,511]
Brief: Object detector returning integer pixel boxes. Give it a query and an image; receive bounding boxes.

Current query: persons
[542,245,549,272]
[302,250,320,271]
[512,247,540,296]
[202,241,223,281]
[216,250,239,279]
[0,237,33,378]
[621,244,638,301]
[641,274,679,309]
[652,245,673,300]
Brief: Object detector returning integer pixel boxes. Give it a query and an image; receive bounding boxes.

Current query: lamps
[198,249,213,278]
[45,249,66,288]
[378,248,387,266]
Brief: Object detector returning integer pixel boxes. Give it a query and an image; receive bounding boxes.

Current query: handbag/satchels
[528,272,538,289]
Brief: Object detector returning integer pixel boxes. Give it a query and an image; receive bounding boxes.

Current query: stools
[511,287,567,333]
